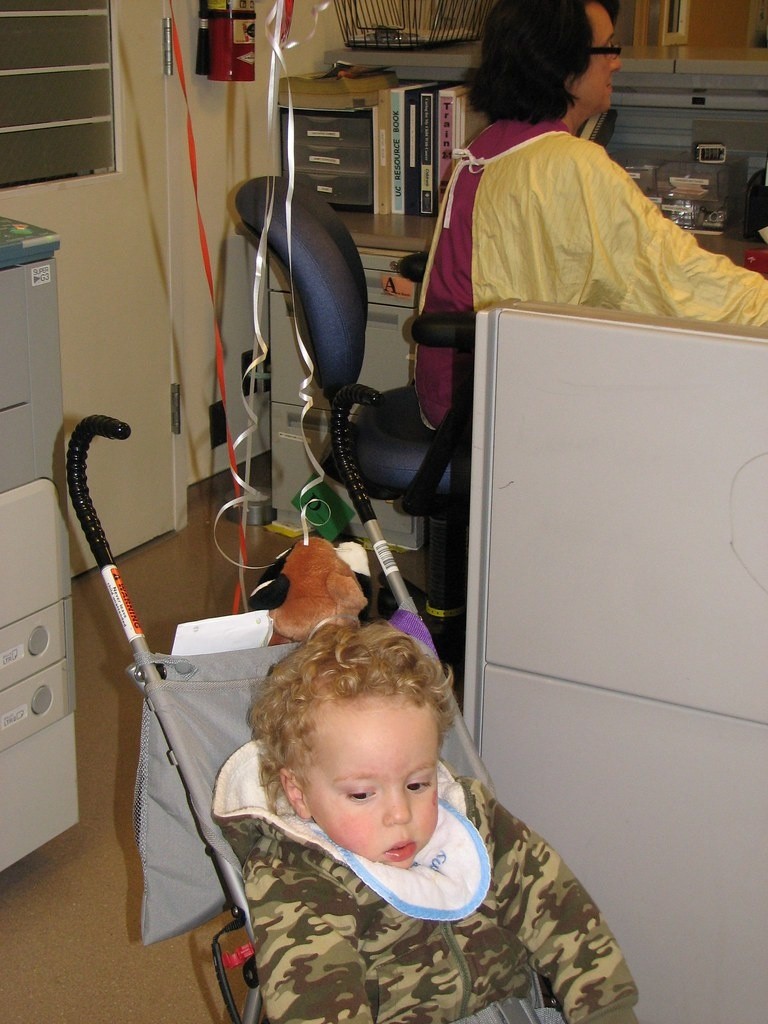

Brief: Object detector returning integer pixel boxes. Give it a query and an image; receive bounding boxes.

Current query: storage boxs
[608,149,749,231]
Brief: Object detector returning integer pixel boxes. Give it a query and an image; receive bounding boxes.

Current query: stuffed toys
[249,537,368,646]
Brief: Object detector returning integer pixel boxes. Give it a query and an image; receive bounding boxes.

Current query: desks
[271,211,768,1024]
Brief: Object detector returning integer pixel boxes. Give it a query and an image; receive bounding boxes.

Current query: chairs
[235,174,476,685]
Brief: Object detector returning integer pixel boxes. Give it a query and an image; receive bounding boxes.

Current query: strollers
[68,383,492,1024]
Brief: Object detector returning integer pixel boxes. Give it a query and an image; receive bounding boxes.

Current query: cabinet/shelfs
[280,108,374,214]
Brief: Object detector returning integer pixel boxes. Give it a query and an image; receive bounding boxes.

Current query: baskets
[334,0,493,52]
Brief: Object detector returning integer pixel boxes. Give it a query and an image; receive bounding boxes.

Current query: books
[279,61,489,219]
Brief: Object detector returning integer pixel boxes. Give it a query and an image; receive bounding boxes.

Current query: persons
[211,615,640,1024]
[412,0,768,428]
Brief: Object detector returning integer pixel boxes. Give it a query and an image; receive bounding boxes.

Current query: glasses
[591,44,622,60]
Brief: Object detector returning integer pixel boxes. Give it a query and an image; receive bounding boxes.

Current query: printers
[0,218,79,874]
